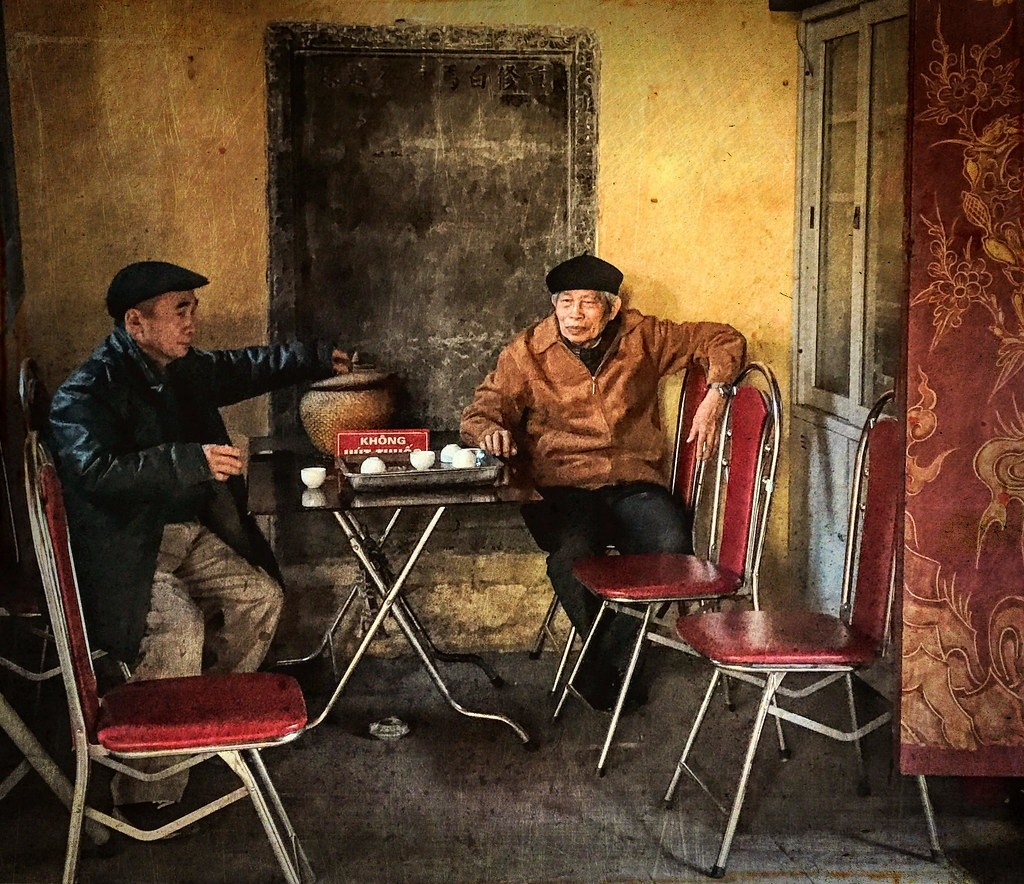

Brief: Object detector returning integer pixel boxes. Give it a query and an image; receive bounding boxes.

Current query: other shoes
[570,666,642,717]
[111,806,200,841]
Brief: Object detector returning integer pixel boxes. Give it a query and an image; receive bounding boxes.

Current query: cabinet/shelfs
[785,0,917,705]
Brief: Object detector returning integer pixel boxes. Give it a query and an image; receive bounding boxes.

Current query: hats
[106,261,210,317]
[546,251,623,296]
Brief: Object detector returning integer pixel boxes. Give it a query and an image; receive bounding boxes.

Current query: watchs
[711,382,733,399]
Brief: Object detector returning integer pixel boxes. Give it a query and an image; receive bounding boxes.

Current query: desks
[243,430,544,755]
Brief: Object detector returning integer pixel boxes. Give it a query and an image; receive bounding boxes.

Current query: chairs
[530,355,947,879]
[0,355,320,884]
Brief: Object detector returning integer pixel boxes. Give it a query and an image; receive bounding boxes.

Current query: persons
[460,252,747,715]
[43,261,350,840]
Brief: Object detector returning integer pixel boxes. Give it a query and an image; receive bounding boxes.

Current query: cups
[440,444,461,462]
[301,467,326,489]
[361,457,387,474]
[452,450,477,469]
[410,451,435,470]
[302,489,328,507]
